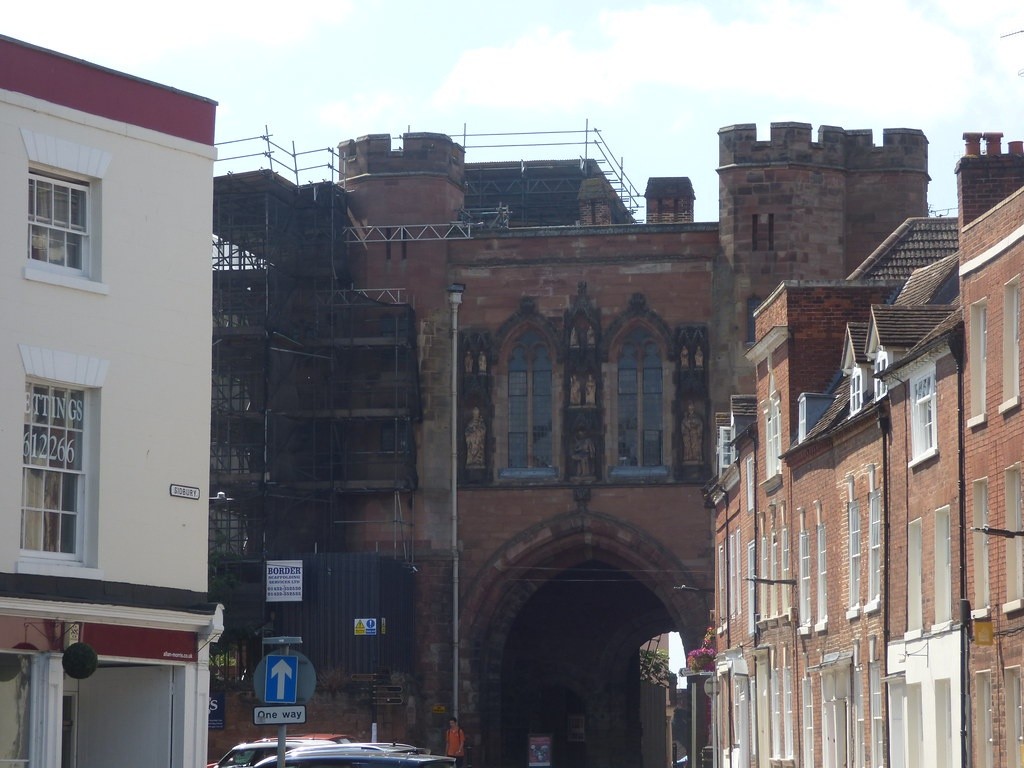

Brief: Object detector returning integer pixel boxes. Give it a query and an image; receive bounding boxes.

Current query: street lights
[445,280,466,726]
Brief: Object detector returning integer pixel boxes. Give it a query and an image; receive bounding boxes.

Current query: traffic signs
[350,673,405,706]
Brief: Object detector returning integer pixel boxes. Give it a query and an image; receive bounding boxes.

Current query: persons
[445,717,465,768]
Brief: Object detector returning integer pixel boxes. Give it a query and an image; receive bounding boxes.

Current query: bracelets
[458,750,460,753]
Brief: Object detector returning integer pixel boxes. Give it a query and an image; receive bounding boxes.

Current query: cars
[208,735,457,768]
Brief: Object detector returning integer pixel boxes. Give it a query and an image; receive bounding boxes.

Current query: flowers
[687,626,718,672]
